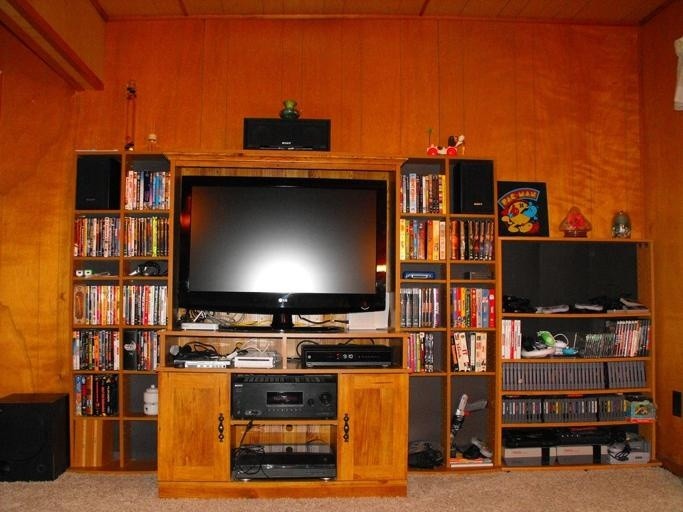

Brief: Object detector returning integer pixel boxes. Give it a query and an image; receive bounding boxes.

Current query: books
[501,319,522,358]
[400,287,441,328]
[74,419,115,468]
[605,318,650,358]
[122,216,168,257]
[122,329,164,371]
[125,170,170,210]
[122,285,168,325]
[450,331,487,372]
[73,285,119,325]
[408,332,434,372]
[73,215,119,257]
[72,330,119,370]
[400,218,445,260]
[450,220,494,260]
[450,287,495,328]
[401,173,446,213]
[75,374,117,416]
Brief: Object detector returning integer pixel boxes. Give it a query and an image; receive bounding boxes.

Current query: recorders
[403,271,436,280]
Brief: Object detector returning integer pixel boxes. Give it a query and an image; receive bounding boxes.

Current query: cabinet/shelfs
[398,154,499,476]
[155,329,409,498]
[500,236,662,470]
[70,144,171,474]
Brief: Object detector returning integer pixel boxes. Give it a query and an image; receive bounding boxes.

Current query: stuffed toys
[427,135,465,155]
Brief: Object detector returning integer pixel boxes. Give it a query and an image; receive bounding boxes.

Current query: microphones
[169,344,188,357]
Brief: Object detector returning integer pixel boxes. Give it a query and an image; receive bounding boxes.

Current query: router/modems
[180,311,219,331]
[234,352,279,368]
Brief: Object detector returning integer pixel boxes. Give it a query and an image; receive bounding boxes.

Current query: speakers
[243,117,332,152]
[0,391,71,482]
[74,156,121,211]
[452,160,495,215]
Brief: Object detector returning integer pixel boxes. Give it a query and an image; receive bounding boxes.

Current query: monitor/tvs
[178,175,388,315]
